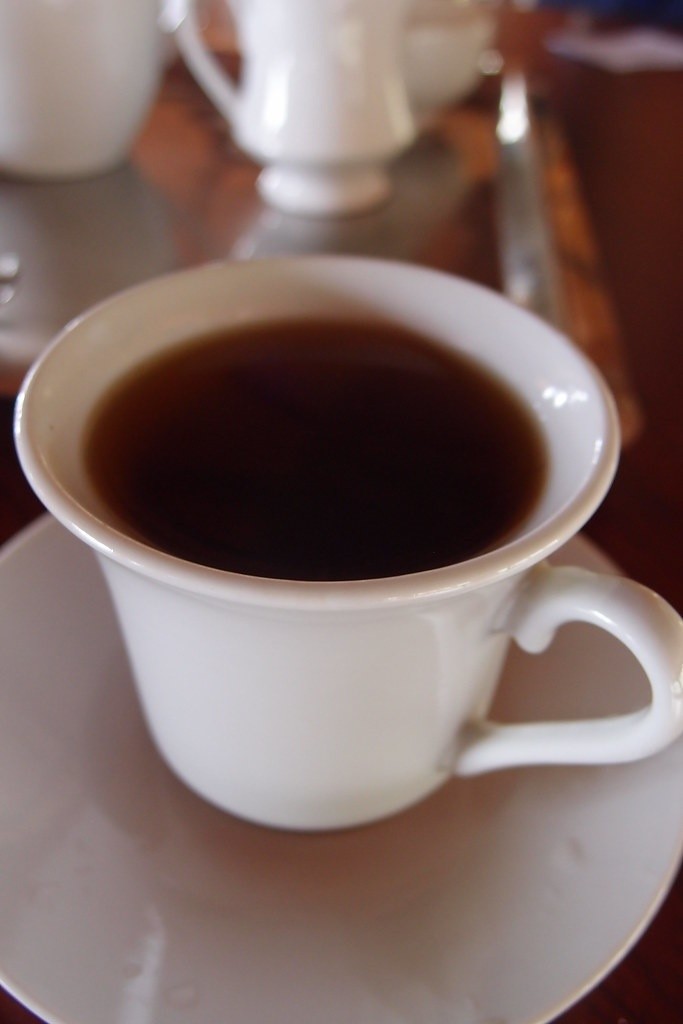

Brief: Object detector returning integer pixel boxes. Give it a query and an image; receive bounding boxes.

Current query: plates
[0,513,683,1024]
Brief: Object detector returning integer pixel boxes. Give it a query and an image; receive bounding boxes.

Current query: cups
[0,1,180,184]
[16,249,683,834]
[158,0,421,213]
[400,16,502,112]
